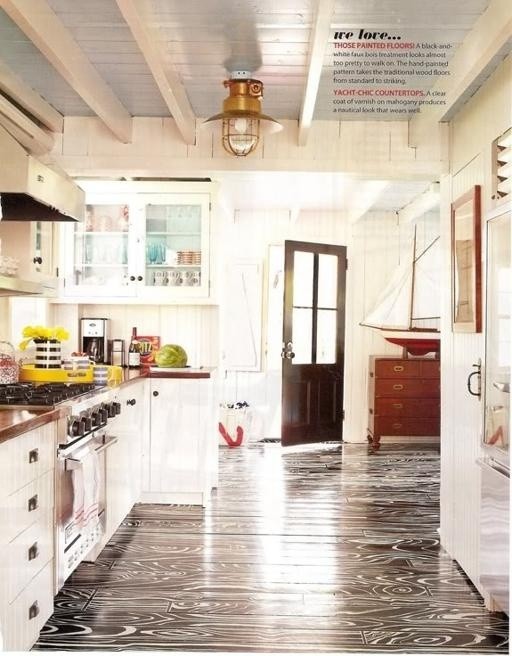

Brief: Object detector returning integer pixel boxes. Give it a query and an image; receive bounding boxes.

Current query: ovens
[57,399,122,589]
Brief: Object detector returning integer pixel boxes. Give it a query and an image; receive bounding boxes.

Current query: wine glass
[80,241,166,265]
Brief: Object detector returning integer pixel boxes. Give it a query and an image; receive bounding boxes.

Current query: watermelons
[154,345,188,368]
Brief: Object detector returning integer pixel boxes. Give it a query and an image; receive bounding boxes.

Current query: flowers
[18,324,71,351]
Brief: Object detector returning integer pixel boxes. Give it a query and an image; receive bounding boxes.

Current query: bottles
[128,327,140,369]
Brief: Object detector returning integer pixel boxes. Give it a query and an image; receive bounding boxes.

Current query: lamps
[202,69,283,158]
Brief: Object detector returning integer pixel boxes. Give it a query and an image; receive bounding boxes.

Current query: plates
[177,251,201,265]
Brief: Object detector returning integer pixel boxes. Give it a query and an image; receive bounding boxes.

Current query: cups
[153,270,201,287]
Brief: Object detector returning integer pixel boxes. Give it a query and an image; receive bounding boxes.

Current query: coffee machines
[81,318,110,365]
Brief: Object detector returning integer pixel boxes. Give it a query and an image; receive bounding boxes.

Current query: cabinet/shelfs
[118,385,142,521]
[0,418,57,651]
[366,354,440,452]
[148,380,216,494]
[0,220,59,288]
[60,192,213,298]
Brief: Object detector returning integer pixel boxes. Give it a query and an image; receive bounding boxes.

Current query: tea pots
[0,341,30,385]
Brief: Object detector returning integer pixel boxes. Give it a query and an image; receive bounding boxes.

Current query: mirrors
[447,184,483,334]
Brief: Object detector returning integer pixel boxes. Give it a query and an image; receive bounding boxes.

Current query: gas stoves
[0,380,105,408]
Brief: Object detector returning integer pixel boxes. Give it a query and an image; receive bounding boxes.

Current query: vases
[32,339,62,369]
